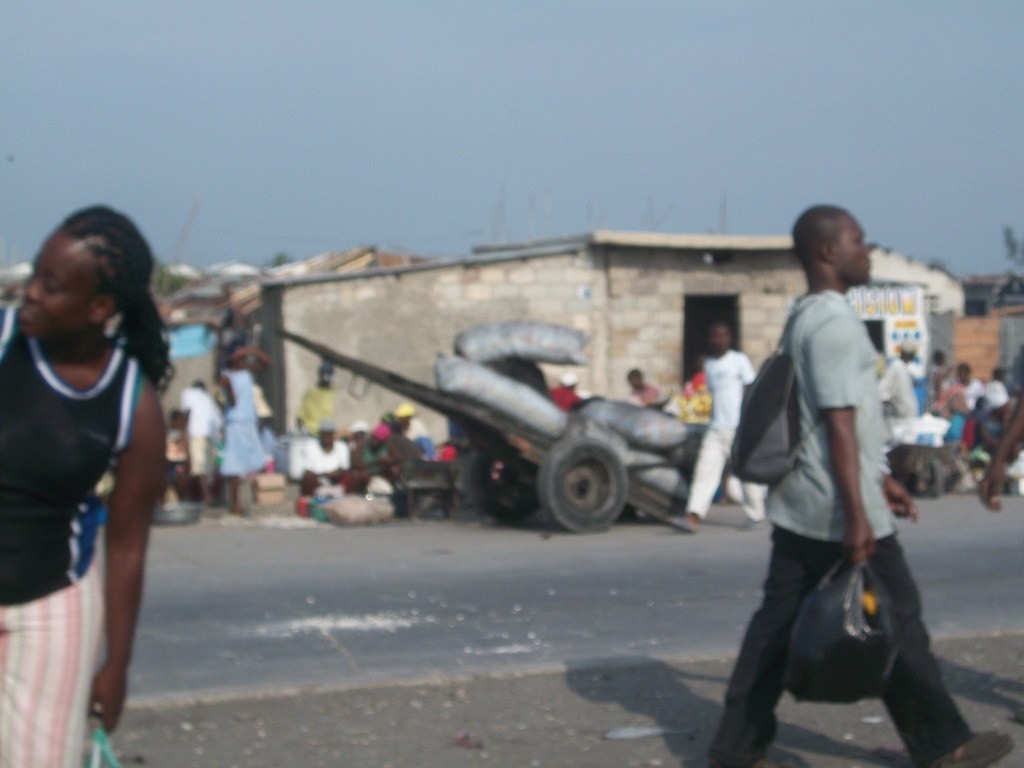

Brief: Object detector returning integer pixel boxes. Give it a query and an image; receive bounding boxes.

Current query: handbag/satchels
[783,557,900,704]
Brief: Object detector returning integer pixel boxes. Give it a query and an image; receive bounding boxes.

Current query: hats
[316,421,337,432]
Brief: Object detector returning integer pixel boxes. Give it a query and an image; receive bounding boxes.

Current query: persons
[666,318,766,532]
[0,205,177,767]
[296,363,433,517]
[878,341,1018,494]
[980,395,1024,721]
[552,375,580,408]
[707,204,1013,767]
[628,368,659,404]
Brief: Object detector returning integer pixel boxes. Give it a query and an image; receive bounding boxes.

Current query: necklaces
[173,341,272,519]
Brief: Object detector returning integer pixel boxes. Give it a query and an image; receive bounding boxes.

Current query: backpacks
[731,293,804,483]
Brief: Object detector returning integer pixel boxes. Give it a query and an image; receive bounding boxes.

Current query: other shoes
[744,517,768,536]
[918,730,1014,768]
[665,516,697,532]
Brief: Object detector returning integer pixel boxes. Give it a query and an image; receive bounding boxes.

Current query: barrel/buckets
[295,495,310,516]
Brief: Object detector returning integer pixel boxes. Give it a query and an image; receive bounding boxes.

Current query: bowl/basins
[151,504,200,524]
